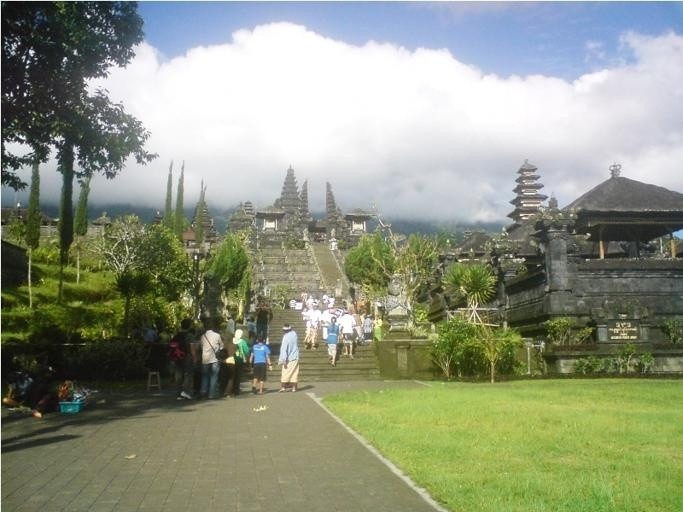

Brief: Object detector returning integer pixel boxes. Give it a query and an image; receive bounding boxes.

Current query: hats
[281,324,291,331]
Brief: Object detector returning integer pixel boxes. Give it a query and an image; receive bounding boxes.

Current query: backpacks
[168,340,186,362]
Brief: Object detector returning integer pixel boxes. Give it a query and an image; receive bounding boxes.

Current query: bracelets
[267,361,272,367]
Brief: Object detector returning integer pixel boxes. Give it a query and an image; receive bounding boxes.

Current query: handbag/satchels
[215,349,229,361]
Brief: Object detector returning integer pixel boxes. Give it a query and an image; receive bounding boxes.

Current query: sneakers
[329,357,336,367]
[252,386,257,395]
[345,353,354,360]
[292,390,296,393]
[279,389,286,392]
[311,347,317,351]
[305,344,308,349]
[177,391,192,401]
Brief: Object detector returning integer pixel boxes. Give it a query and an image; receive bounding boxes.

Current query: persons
[167,313,247,400]
[247,316,255,337]
[300,291,372,367]
[277,323,300,393]
[248,337,273,393]
[255,295,272,344]
[289,297,296,310]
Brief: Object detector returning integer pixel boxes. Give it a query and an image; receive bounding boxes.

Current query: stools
[147,370,161,390]
